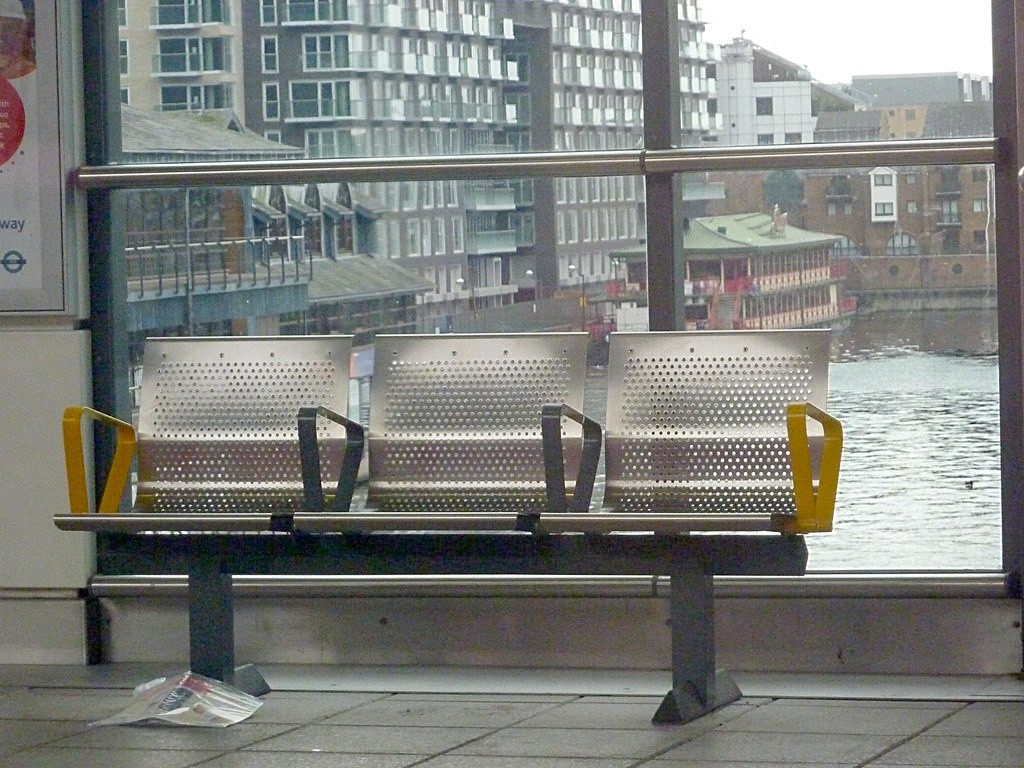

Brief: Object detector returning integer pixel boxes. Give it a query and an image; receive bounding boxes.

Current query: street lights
[611,261,626,291]
[526,269,543,330]
[569,265,585,320]
[456,278,476,320]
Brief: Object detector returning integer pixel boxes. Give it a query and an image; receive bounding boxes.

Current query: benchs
[53,328,843,726]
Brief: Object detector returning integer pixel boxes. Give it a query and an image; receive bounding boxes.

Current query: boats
[585,202,858,366]
